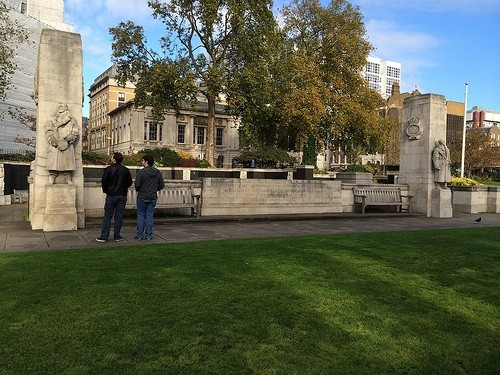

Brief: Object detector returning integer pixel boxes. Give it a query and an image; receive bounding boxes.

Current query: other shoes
[96,238,107,242]
[134,236,139,239]
[145,237,152,240]
[113,237,123,242]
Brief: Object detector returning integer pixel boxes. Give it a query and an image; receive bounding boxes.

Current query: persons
[432,138,452,189]
[217,153,240,168]
[96,151,133,242]
[134,155,164,240]
[45,103,79,185]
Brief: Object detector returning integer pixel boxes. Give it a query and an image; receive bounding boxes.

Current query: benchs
[352,187,413,215]
[124,183,201,219]
